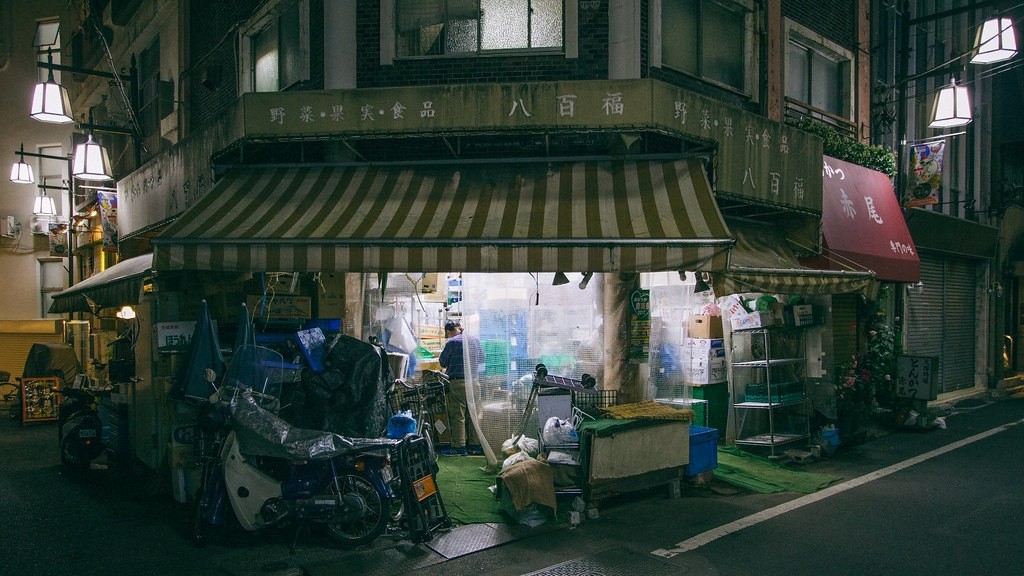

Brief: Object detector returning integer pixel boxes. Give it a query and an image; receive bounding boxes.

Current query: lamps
[908,279,923,295]
[968,207,1007,219]
[551,271,572,285]
[933,199,976,210]
[692,271,710,293]
[988,282,1003,297]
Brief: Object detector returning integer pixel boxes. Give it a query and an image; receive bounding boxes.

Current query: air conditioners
[30,215,56,235]
[0,216,14,236]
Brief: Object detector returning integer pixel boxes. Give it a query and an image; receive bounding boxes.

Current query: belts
[449,377,464,381]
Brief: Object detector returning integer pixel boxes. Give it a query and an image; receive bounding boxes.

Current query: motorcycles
[192,344,404,555]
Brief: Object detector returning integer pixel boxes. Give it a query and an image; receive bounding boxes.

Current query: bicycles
[0,374,23,411]
[387,367,450,444]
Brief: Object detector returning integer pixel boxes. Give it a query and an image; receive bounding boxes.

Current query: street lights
[8,142,74,345]
[891,0,1019,359]
[29,46,142,186]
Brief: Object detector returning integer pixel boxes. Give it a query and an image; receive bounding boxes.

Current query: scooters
[49,369,110,475]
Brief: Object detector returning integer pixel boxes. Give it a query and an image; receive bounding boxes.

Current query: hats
[444,321,461,339]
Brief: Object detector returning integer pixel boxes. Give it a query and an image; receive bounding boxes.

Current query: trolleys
[510,363,598,446]
[395,431,451,545]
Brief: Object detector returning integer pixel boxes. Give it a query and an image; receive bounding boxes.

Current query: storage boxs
[683,426,721,476]
[86,327,134,456]
[650,280,827,435]
[148,270,602,503]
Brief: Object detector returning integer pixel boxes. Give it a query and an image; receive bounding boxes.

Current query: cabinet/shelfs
[731,326,812,458]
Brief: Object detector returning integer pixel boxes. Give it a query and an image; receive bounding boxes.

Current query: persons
[438,321,484,456]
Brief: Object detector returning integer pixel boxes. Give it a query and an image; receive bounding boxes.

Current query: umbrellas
[173,297,226,400]
[230,301,265,392]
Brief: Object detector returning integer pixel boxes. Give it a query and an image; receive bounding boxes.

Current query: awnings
[47,255,152,314]
[712,221,876,300]
[151,162,731,273]
[818,154,921,283]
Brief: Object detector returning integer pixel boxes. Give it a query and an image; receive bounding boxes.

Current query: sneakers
[440,447,467,456]
[466,445,483,455]
